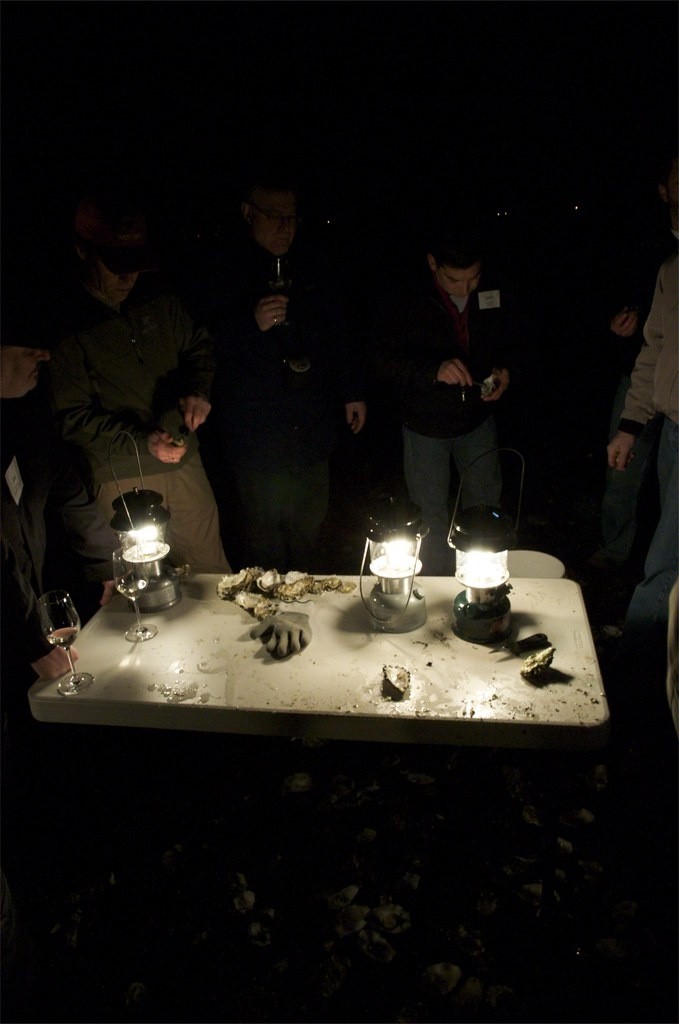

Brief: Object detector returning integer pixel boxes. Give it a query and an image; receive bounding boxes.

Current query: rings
[274,316,277,323]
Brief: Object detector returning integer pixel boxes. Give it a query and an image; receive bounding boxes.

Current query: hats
[74,194,159,275]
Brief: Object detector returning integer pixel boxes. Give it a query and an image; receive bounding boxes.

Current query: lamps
[446,450,525,643]
[106,429,183,610]
[361,490,427,633]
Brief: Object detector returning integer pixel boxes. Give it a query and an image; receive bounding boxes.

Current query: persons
[41,196,234,573]
[198,173,368,574]
[381,230,542,580]
[1,292,127,702]
[578,153,678,752]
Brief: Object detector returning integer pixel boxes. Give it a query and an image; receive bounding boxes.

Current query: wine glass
[112,547,159,642]
[38,589,96,696]
[267,257,292,327]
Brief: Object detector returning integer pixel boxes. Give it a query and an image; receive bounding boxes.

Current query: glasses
[248,201,299,225]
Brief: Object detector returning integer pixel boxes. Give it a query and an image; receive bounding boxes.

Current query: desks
[28,573,612,752]
